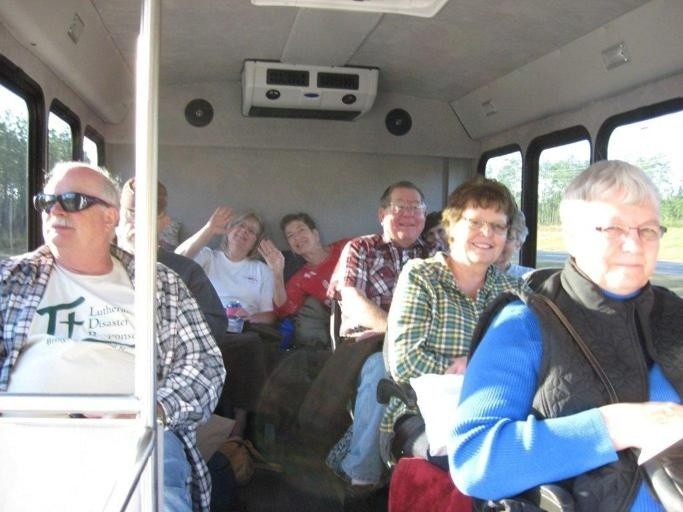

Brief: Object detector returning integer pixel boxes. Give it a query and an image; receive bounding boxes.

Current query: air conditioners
[240,60,379,122]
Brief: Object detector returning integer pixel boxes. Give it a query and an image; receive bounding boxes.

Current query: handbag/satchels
[218,436,255,485]
[206,451,234,512]
[325,424,397,489]
[630,440,683,511]
[293,297,330,346]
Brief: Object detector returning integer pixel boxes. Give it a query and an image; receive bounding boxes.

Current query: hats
[121,176,167,216]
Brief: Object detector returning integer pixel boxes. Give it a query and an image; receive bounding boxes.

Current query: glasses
[595,225,666,241]
[237,222,258,238]
[33,191,110,211]
[464,217,508,234]
[388,202,426,212]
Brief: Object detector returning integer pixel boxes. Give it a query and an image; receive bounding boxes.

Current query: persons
[379,179,528,476]
[1,159,229,512]
[324,177,434,510]
[256,214,355,321]
[114,171,228,359]
[442,157,683,512]
[422,209,447,249]
[491,208,535,279]
[172,202,277,449]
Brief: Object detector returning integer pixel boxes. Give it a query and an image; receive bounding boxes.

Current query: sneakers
[344,484,376,511]
